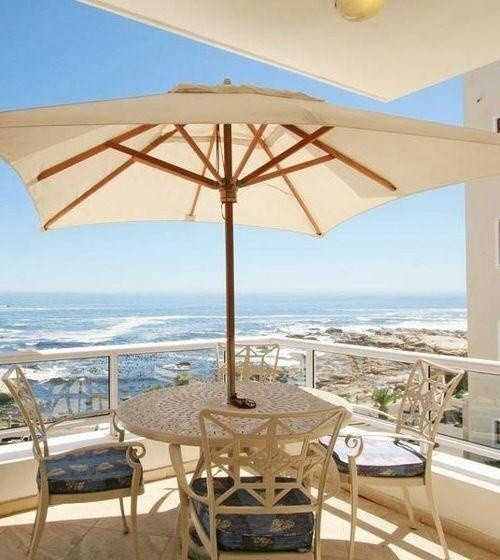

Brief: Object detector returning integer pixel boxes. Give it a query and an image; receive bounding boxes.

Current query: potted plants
[370,387,402,420]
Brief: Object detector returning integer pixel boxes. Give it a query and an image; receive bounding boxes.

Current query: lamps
[335,0,389,23]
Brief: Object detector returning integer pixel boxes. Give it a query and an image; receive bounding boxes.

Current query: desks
[116,383,352,473]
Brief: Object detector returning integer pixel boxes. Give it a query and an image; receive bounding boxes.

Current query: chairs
[2,364,146,560]
[318,359,465,560]
[169,406,347,560]
[216,342,280,383]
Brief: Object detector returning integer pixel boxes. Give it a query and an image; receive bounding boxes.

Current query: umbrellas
[0,76,500,405]
